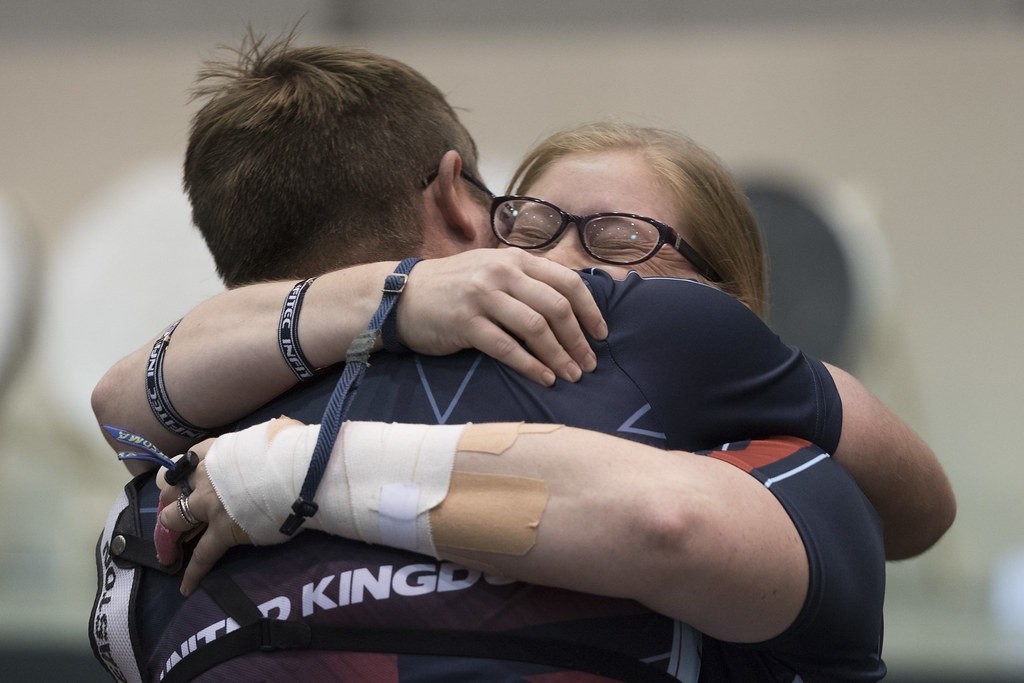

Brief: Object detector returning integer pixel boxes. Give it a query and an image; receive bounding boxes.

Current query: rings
[177,496,200,527]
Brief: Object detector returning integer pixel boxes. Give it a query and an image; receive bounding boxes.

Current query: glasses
[489,196,724,285]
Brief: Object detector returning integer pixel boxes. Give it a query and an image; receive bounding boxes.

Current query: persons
[88,46,957,683]
